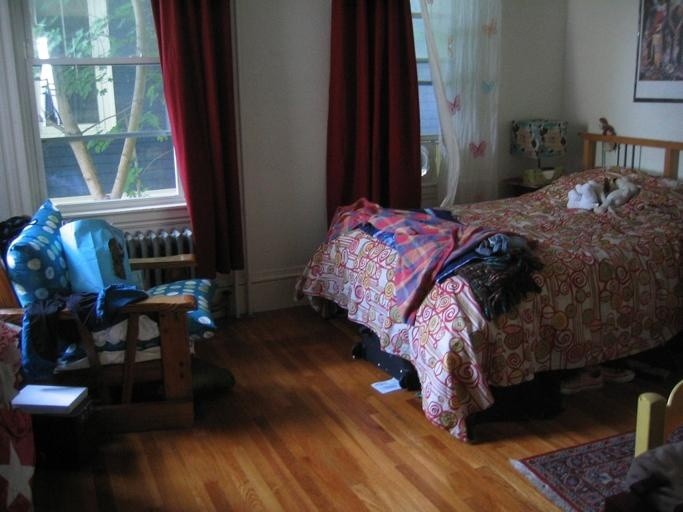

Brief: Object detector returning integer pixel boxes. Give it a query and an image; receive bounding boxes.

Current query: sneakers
[603,364,635,383]
[559,368,604,395]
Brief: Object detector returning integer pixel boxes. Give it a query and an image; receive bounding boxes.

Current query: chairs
[0,214,195,434]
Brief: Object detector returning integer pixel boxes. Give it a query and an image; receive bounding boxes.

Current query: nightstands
[503,177,545,198]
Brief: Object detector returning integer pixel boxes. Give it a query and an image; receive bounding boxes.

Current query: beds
[604,379,683,511]
[294,133,682,443]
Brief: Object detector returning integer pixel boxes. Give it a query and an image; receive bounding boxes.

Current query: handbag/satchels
[604,440,682,512]
[59,218,133,292]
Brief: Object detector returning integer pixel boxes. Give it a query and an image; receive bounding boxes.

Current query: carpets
[509,430,635,511]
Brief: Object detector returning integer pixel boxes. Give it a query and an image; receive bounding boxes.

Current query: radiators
[123,228,195,292]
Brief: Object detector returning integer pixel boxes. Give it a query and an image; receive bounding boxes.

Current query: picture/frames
[633,0,683,103]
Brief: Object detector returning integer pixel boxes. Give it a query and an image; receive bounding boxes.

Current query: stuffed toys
[565,179,602,213]
[598,118,617,152]
[593,173,638,216]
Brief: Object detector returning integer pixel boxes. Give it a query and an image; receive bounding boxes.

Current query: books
[10,382,87,417]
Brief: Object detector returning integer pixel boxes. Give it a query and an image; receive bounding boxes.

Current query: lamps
[510,118,568,187]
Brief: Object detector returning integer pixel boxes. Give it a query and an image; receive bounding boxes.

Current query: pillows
[5,199,71,379]
[145,278,218,334]
